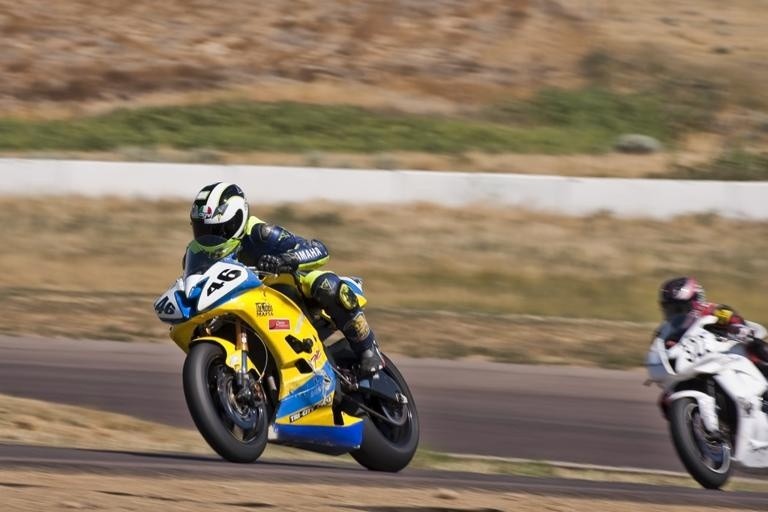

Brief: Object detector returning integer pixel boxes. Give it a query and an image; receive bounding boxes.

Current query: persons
[181,181,386,380]
[658,275,768,410]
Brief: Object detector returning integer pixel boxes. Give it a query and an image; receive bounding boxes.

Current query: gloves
[257,256,284,272]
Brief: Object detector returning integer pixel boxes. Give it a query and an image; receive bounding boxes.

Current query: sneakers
[356,341,385,375]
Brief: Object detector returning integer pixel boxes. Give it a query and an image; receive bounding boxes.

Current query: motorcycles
[150,237,422,475]
[642,313,767,490]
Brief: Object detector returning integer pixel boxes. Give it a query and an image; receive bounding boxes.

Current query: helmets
[661,277,698,313]
[190,182,248,259]
